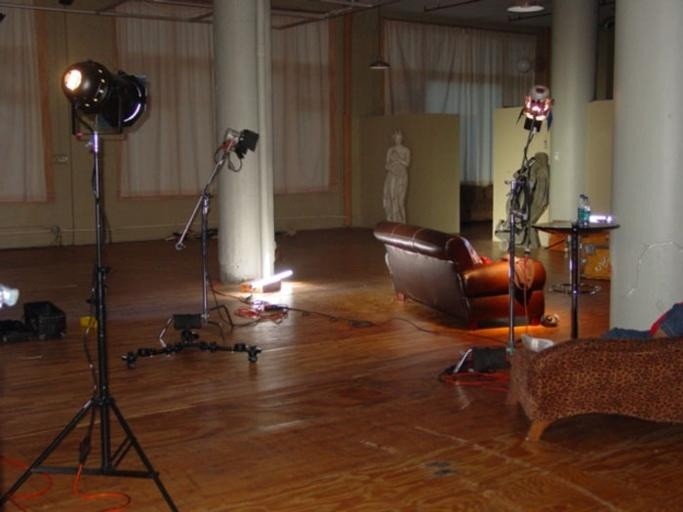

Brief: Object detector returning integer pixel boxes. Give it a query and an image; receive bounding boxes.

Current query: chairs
[505,302,683,441]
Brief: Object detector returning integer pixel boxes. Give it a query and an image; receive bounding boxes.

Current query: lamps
[61,61,149,129]
[515,84,555,133]
[223,128,259,159]
[506,1,545,13]
[367,4,389,70]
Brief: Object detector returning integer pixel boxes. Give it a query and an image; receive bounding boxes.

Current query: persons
[381,130,411,224]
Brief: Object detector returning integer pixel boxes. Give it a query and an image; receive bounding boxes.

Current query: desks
[531,214,621,339]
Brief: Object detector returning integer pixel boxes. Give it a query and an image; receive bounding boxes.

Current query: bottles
[577,194,590,226]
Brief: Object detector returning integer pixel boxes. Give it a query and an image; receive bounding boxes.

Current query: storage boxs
[23,300,66,341]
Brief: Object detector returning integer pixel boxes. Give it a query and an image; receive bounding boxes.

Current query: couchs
[373,220,546,330]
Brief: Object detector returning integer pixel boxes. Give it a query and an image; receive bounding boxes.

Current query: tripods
[0,118,178,512]
[158,152,234,339]
[453,179,528,373]
